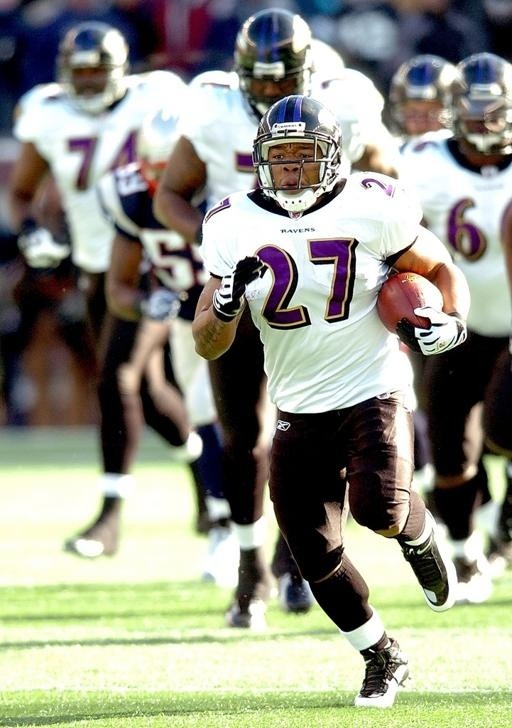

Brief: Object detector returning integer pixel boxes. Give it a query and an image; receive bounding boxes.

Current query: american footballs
[376,273,444,334]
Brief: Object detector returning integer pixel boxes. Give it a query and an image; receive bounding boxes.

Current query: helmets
[234,8,312,117]
[388,55,453,134]
[445,53,512,156]
[136,104,207,208]
[252,95,352,213]
[57,21,130,114]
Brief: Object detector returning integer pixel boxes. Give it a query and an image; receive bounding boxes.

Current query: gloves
[18,228,71,270]
[396,307,468,357]
[213,255,268,322]
[140,288,189,321]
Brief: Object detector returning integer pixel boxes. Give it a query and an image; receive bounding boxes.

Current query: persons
[190,90,471,707]
[1,0,509,633]
